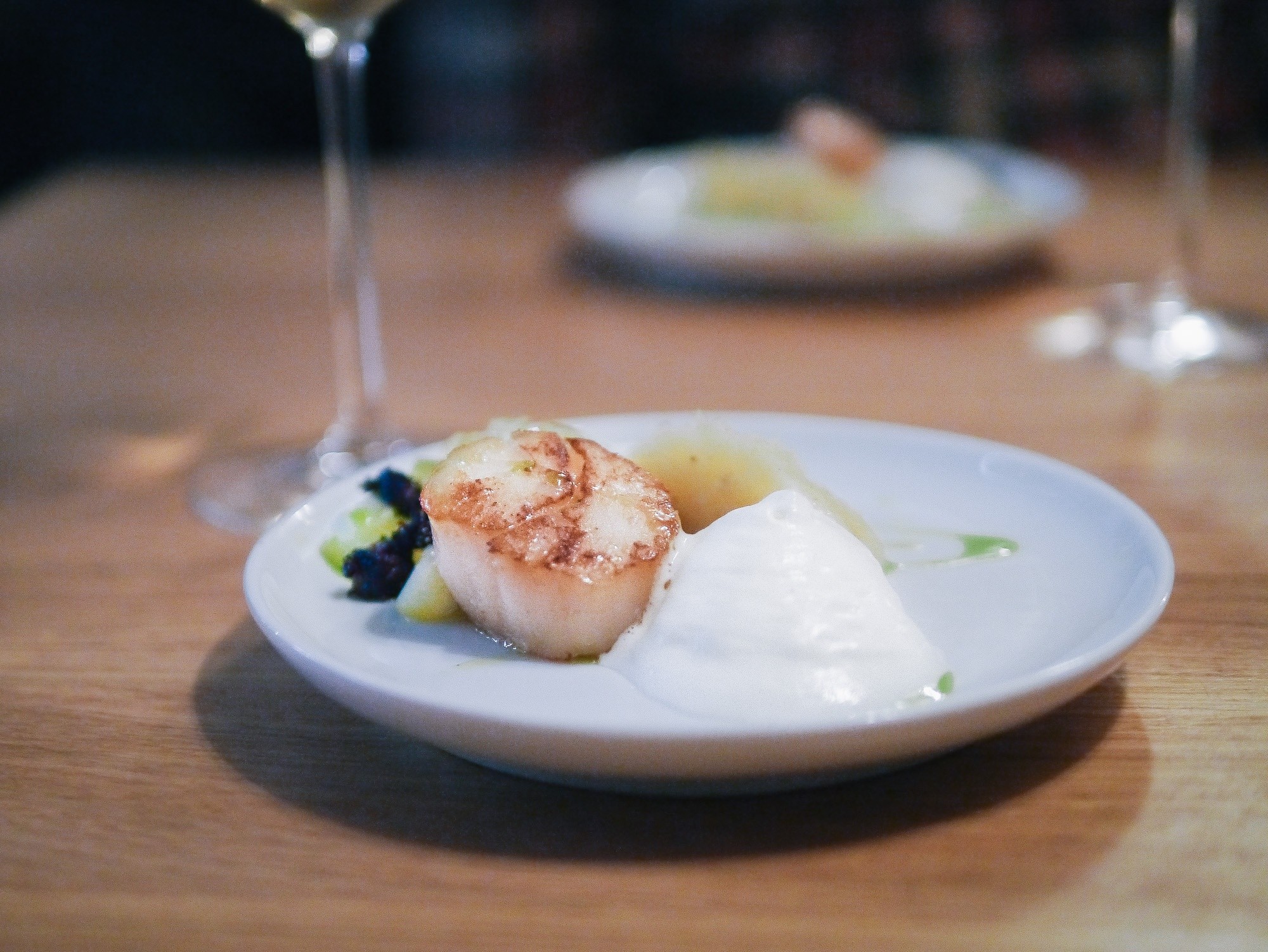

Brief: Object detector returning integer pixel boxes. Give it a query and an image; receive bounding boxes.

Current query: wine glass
[191,3,439,536]
[1027,1,1267,367]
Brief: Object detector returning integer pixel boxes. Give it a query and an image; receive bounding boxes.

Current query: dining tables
[1,156,1268,948]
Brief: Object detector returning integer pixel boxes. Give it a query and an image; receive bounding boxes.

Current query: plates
[557,140,1083,286]
[248,413,1175,793]
